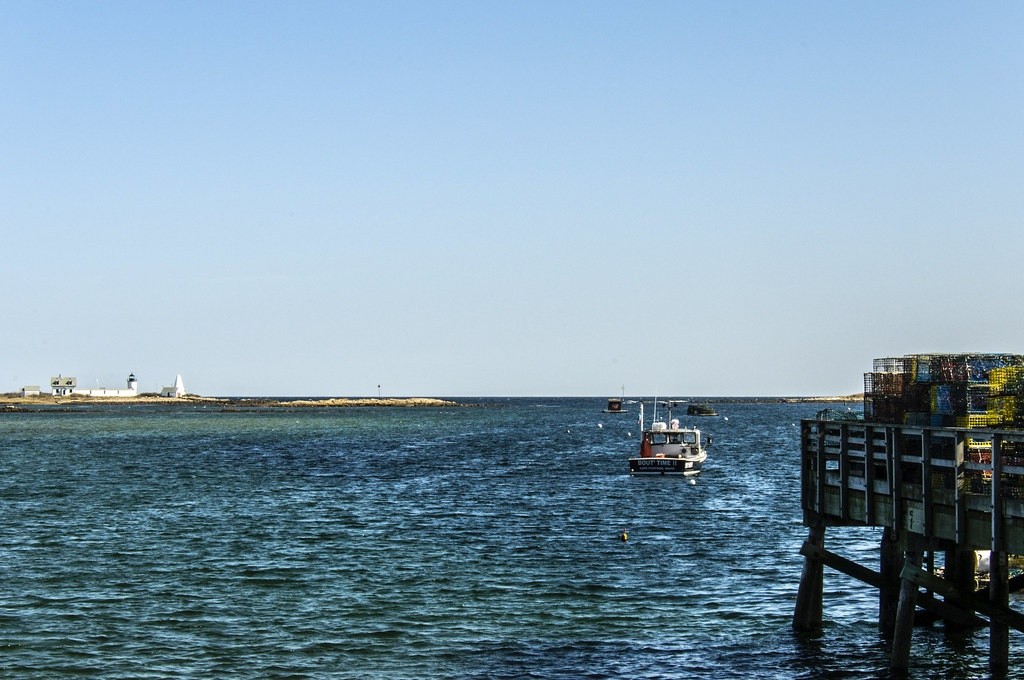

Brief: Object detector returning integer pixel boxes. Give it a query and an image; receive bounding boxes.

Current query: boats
[602,398,629,413]
[627,392,712,476]
[687,401,720,417]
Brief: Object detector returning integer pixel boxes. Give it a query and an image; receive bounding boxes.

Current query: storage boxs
[864,353,1024,498]
